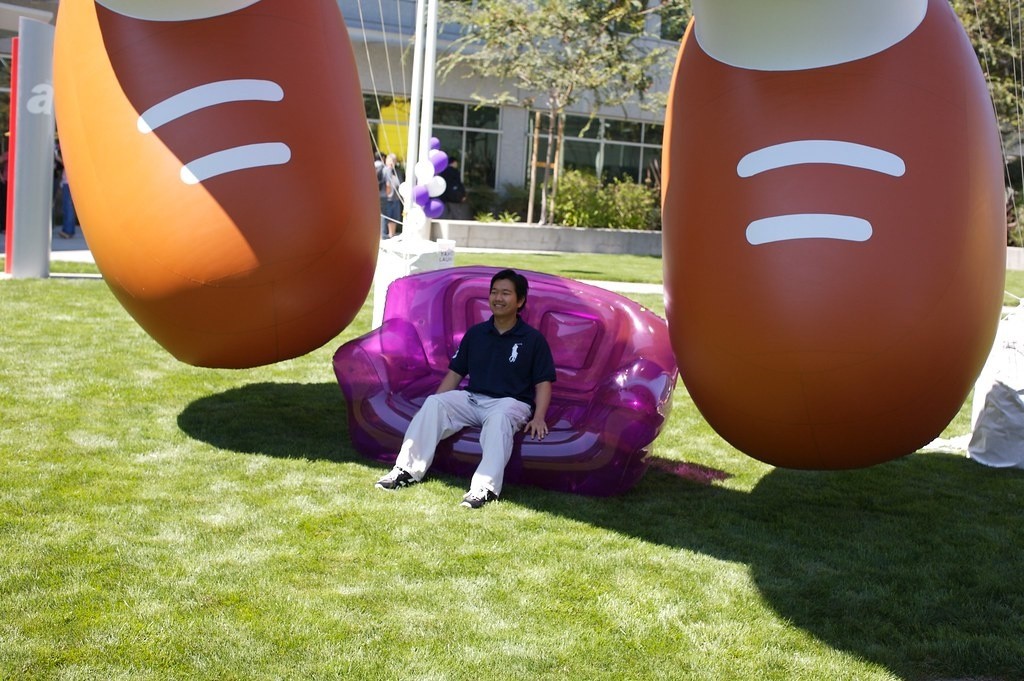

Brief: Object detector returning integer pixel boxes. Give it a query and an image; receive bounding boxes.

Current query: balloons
[398,138,450,232]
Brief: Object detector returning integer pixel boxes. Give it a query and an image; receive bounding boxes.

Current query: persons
[373,151,398,238]
[440,157,466,203]
[376,269,557,511]
[53,138,76,238]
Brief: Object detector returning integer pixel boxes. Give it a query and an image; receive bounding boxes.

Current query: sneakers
[460,485,495,509]
[375,468,417,492]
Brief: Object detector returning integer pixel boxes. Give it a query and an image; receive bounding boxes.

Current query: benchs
[333,265,678,499]
